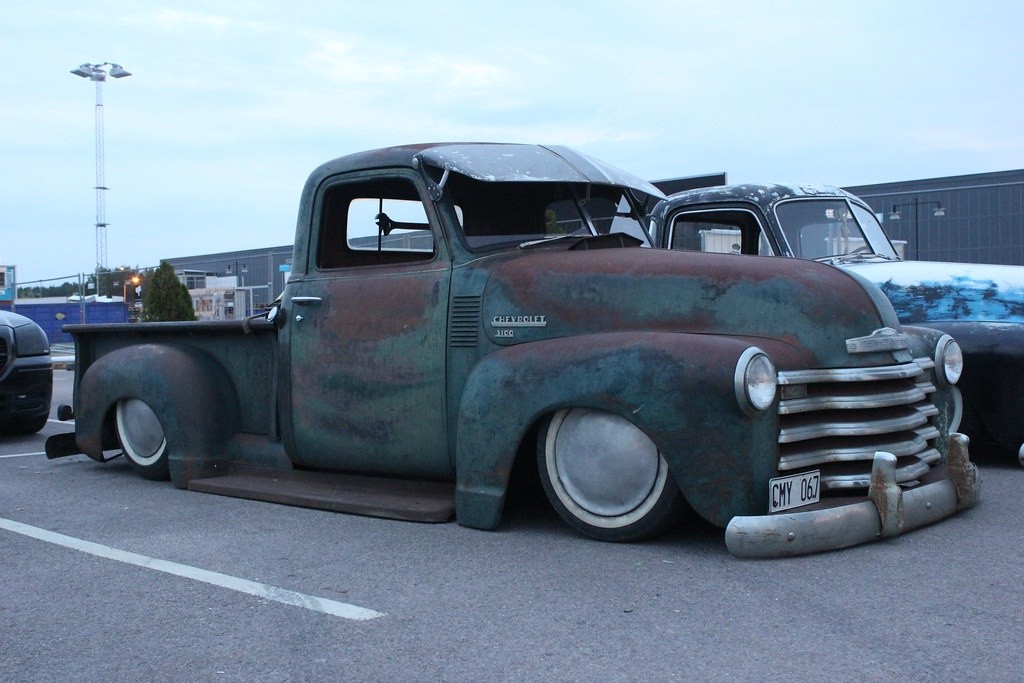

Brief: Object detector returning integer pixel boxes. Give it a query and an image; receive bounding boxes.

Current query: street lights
[69,61,133,296]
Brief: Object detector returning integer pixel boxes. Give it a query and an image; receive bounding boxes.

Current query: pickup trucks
[46,140,983,560]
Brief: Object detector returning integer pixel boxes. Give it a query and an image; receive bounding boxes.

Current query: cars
[1,306,54,437]
[642,183,1023,467]
